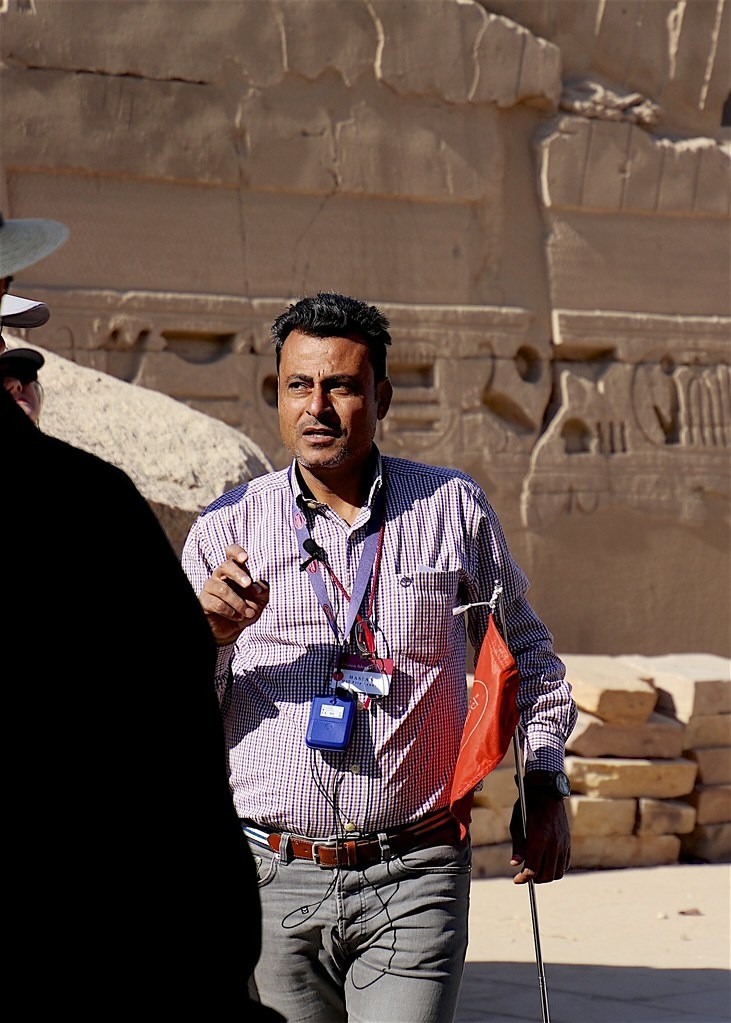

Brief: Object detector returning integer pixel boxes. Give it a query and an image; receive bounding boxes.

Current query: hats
[0,348,45,379]
[0,295,50,329]
[0,211,68,278]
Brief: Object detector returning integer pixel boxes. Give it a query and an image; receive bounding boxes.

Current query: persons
[1,207,286,1023]
[183,293,575,1022]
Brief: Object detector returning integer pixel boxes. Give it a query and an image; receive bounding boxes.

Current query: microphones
[303,538,324,560]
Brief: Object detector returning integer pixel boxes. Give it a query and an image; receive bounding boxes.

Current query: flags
[449,613,521,842]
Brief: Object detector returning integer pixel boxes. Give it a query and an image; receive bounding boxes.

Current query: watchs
[523,770,571,799]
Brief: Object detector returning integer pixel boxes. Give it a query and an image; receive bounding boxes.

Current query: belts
[243,808,452,869]
